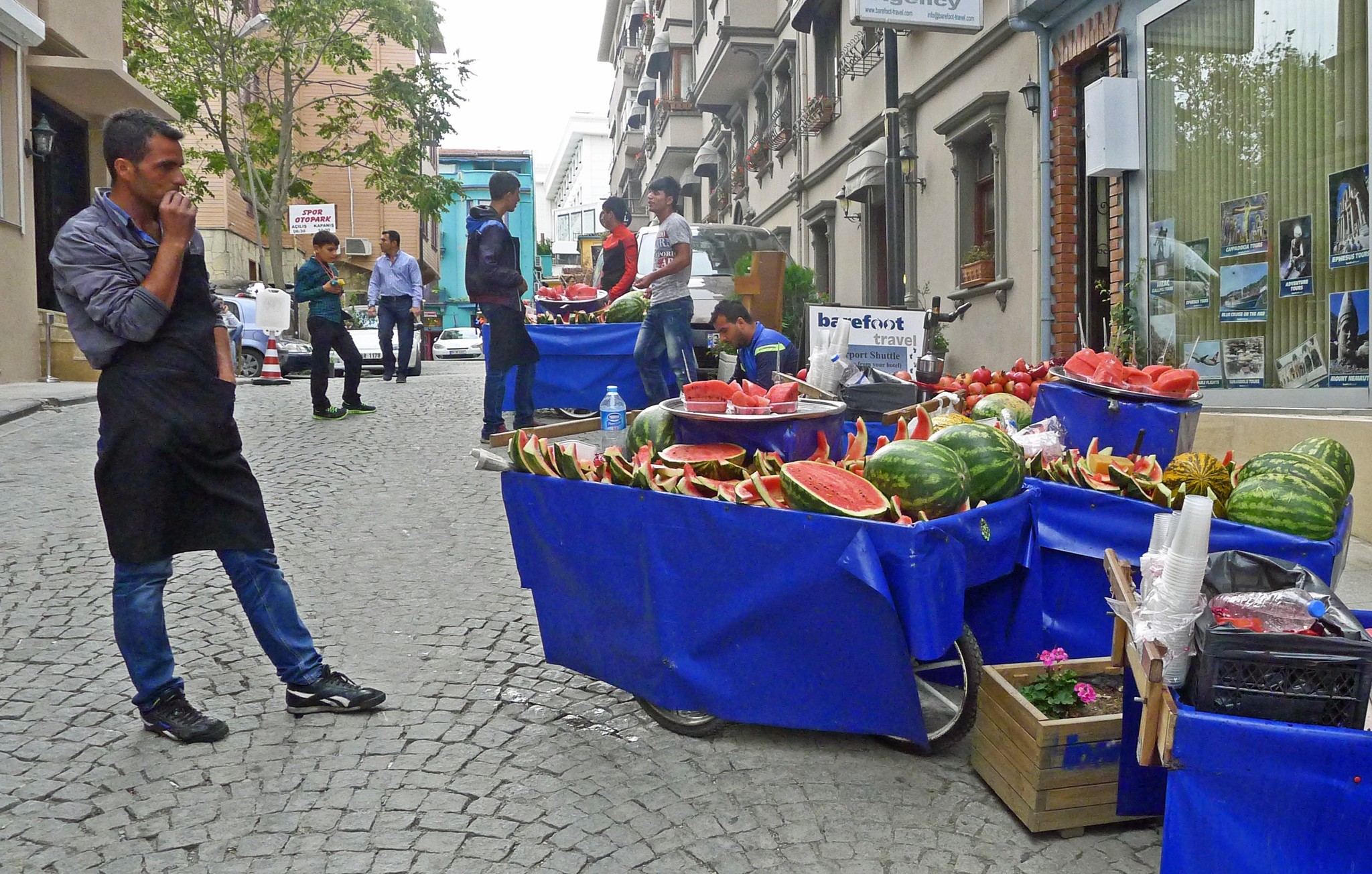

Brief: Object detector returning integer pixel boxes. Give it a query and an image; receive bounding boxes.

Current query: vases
[731,179,744,194]
[808,108,834,133]
[750,149,768,168]
[661,100,692,111]
[637,158,646,164]
[972,656,1162,840]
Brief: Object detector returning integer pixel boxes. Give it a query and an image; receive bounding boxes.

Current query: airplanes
[1187,350,1221,367]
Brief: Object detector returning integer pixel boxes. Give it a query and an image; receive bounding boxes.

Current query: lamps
[899,144,926,194]
[24,113,58,163]
[835,185,861,222]
[1018,73,1040,117]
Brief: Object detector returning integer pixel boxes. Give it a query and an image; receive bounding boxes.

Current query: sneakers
[342,401,377,414]
[284,664,387,713]
[138,687,230,743]
[383,369,396,381]
[312,406,349,420]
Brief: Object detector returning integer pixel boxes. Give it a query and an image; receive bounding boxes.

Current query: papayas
[1163,452,1230,506]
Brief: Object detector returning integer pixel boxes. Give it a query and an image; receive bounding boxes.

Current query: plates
[534,289,608,303]
[659,397,847,422]
[1049,366,1204,400]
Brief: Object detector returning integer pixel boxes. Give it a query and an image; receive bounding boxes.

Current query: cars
[215,288,314,378]
[431,327,485,362]
[328,305,424,377]
[590,222,820,371]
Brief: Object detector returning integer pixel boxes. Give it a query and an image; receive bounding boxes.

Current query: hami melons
[929,412,974,433]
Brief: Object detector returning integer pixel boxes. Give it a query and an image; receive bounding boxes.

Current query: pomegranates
[894,357,1066,409]
[797,368,807,381]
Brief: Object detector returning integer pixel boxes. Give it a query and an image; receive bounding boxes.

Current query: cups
[807,318,851,392]
[1139,495,1213,688]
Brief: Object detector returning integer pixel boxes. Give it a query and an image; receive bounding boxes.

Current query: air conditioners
[345,237,372,256]
[534,256,543,292]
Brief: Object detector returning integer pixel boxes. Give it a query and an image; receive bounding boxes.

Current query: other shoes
[480,423,509,444]
[513,421,548,430]
[395,372,407,383]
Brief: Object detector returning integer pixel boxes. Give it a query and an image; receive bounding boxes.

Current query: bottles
[1207,588,1327,634]
[831,355,872,386]
[599,385,627,451]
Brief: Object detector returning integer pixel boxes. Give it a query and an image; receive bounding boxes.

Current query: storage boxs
[1032,380,1203,470]
[1175,550,1372,729]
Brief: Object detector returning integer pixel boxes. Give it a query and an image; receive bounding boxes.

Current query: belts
[381,295,411,300]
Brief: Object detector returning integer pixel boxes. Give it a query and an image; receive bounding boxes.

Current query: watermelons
[536,282,597,301]
[627,405,677,460]
[778,460,890,523]
[682,379,798,415]
[970,393,1034,434]
[1288,435,1355,499]
[863,439,970,521]
[605,291,652,323]
[1225,473,1336,540]
[1236,451,1347,518]
[927,423,1024,507]
[658,441,746,480]
[1063,347,1199,399]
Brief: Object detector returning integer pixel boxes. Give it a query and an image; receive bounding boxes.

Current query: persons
[560,176,699,409]
[1154,200,1264,257]
[292,231,377,421]
[465,172,546,444]
[49,108,388,746]
[707,300,808,389]
[1332,294,1361,363]
[366,230,425,384]
[1283,226,1305,280]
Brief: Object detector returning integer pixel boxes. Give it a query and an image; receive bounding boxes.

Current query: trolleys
[488,408,985,759]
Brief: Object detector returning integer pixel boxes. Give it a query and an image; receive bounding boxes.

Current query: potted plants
[912,281,950,375]
[715,191,728,210]
[961,245,996,285]
[706,337,739,381]
[773,121,791,148]
[644,136,656,151]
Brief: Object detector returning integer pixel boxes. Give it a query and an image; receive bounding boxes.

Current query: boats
[1224,291,1265,310]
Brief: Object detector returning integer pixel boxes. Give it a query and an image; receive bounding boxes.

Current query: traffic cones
[251,330,292,386]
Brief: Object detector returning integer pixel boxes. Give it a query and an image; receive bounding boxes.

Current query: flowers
[635,152,645,159]
[734,167,744,182]
[1028,644,1102,711]
[745,133,767,169]
[806,95,832,117]
[654,91,683,108]
